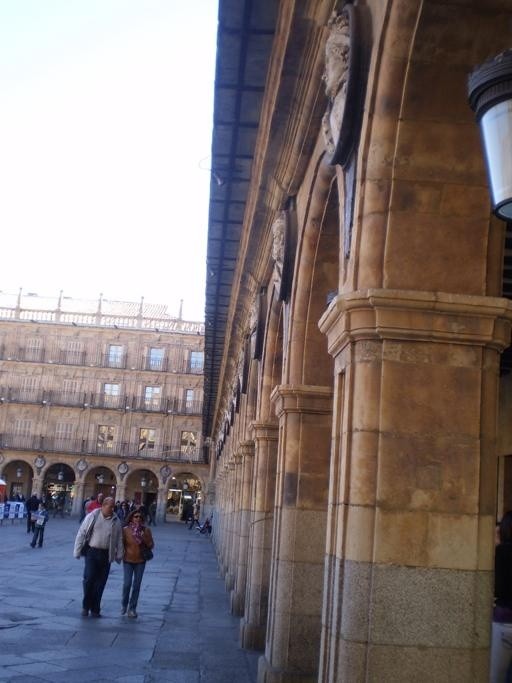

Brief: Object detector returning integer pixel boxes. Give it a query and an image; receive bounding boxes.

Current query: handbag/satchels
[80,541,92,557]
[140,525,154,560]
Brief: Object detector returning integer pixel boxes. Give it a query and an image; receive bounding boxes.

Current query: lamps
[464,50,511,223]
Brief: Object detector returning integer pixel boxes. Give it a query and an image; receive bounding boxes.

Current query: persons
[73,497,125,617]
[183,501,201,530]
[79,492,158,526]
[26,491,41,533]
[30,502,50,548]
[116,509,155,617]
[15,490,25,502]
[53,496,64,519]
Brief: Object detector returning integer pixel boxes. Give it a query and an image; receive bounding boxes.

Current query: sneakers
[80,606,102,618]
[121,604,138,617]
